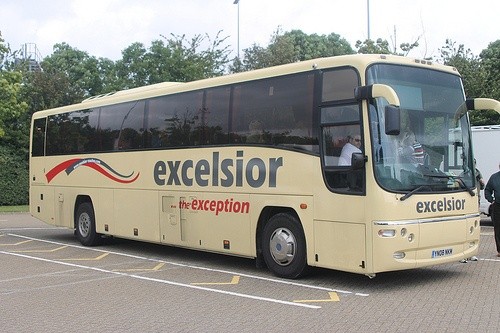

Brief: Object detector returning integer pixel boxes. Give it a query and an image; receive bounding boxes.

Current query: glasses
[352,139,362,143]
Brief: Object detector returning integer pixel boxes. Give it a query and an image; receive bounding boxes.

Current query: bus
[29,54,500,280]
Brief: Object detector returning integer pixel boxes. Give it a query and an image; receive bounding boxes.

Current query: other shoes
[459,259,468,263]
[469,256,478,260]
[495,253,500,257]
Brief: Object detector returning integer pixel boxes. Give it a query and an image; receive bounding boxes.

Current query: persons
[396,127,425,168]
[458,158,485,263]
[338,134,362,167]
[484,159,500,258]
[244,117,306,145]
